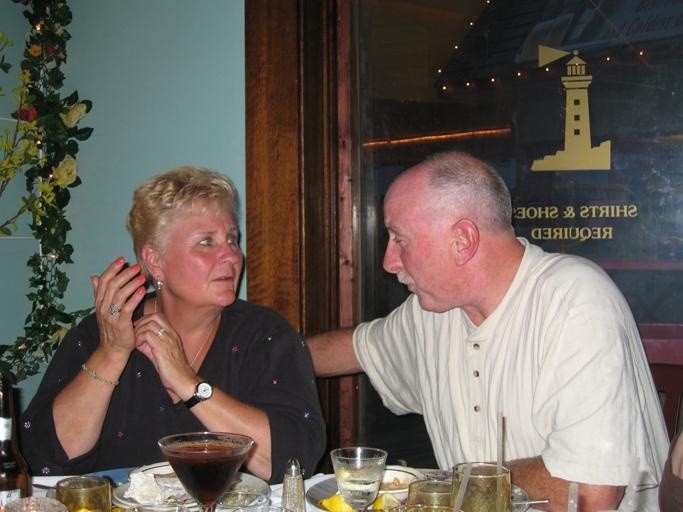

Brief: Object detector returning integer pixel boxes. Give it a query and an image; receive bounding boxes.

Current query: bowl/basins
[379,465,425,502]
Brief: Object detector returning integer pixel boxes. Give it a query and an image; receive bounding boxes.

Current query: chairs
[647,363,682,443]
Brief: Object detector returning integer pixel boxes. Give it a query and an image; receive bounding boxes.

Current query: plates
[306,468,533,511]
[129,462,177,482]
[112,472,273,512]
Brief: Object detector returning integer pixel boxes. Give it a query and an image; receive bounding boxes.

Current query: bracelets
[81,364,120,387]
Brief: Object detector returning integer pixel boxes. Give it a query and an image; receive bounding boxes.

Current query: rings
[157,328,166,337]
[109,303,121,316]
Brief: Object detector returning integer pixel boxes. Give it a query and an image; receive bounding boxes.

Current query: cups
[214,490,268,512]
[3,496,69,512]
[407,479,453,511]
[56,476,112,511]
[452,463,512,511]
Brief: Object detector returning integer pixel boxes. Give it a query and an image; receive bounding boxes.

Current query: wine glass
[157,432,254,512]
[330,447,387,512]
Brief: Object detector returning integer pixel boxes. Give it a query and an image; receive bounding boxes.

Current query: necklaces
[154,296,218,368]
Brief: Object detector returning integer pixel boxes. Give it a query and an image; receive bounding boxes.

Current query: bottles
[0,360,31,512]
[281,456,305,512]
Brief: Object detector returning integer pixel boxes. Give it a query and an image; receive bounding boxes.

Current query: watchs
[183,380,216,410]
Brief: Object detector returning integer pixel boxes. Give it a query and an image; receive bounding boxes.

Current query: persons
[15,168,326,485]
[306,150,672,512]
